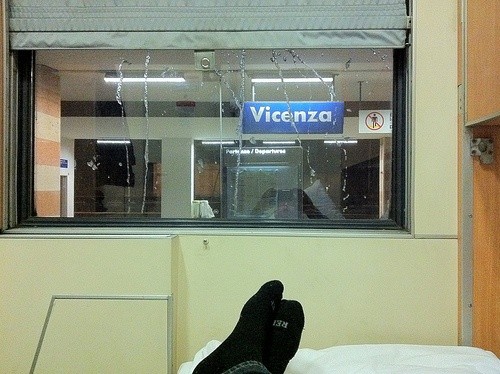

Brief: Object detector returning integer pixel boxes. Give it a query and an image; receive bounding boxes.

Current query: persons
[191,279,306,373]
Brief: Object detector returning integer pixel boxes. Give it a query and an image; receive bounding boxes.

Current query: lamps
[262,141,297,146]
[101,71,185,85]
[96,139,131,146]
[201,140,238,146]
[324,139,358,145]
[251,70,335,83]
